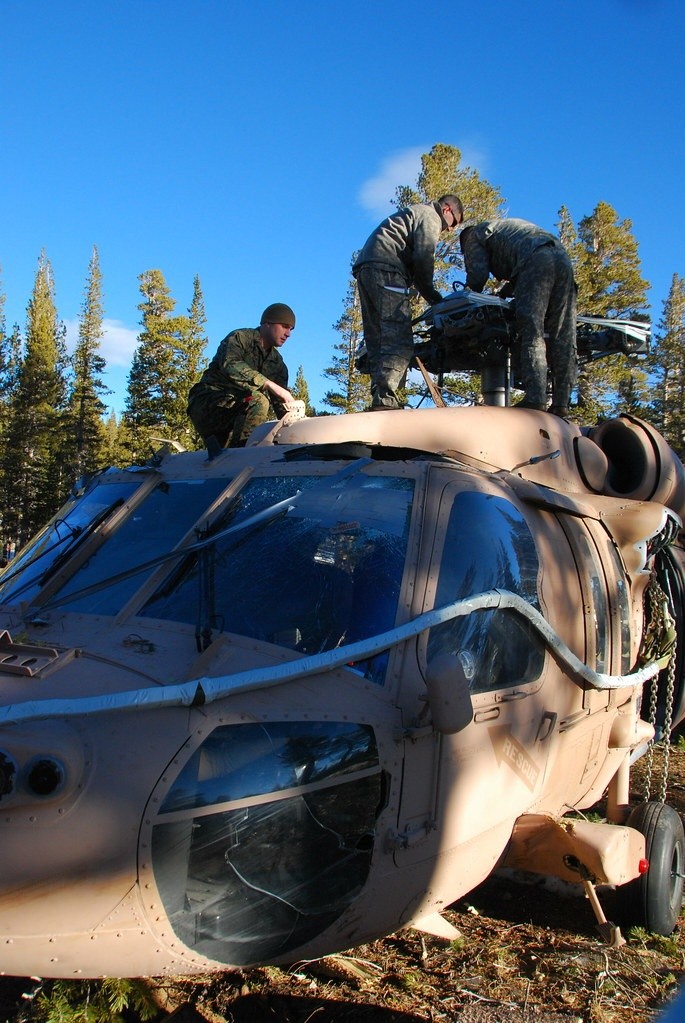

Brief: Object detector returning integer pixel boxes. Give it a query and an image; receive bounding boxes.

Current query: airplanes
[0,271,685,980]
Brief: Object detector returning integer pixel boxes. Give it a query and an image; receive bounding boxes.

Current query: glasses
[445,202,458,228]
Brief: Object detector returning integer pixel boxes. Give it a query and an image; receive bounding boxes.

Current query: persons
[351,194,466,410]
[459,218,578,416]
[2,540,15,568]
[188,302,297,459]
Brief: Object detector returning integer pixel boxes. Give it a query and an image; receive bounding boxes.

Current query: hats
[260,303,295,329]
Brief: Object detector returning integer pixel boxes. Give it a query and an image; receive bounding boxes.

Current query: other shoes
[512,399,546,411]
[547,405,568,417]
